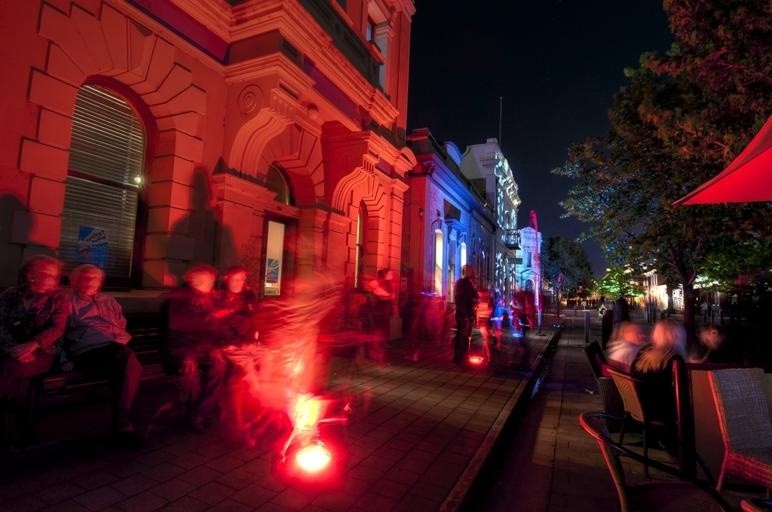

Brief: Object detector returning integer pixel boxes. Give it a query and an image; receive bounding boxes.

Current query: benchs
[23,310,171,449]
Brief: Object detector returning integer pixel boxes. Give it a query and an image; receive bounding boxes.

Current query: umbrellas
[669,114,771,206]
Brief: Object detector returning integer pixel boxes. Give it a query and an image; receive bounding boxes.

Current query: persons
[472,277,538,365]
[599,292,733,448]
[450,263,480,363]
[0,253,71,464]
[159,254,452,475]
[58,261,147,442]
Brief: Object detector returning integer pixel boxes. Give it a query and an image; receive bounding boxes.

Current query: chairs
[578,342,772,512]
